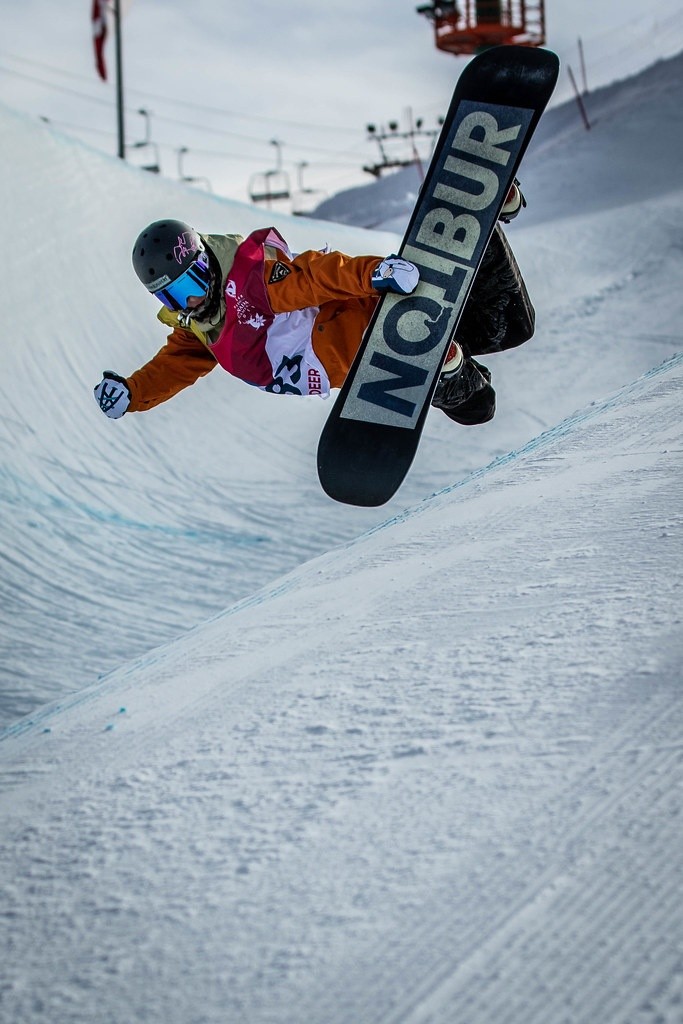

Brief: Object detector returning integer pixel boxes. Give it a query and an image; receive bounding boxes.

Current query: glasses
[154,252,211,312]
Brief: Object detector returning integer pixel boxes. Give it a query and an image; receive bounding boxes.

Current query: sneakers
[442,339,463,379]
[502,182,521,214]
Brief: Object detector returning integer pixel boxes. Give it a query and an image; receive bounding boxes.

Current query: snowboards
[317,46,559,505]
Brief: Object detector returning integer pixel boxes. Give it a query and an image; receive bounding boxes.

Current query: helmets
[132,219,205,294]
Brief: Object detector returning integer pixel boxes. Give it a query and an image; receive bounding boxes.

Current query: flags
[90,0,110,82]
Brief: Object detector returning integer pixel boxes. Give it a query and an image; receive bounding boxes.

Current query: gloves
[372,255,420,296]
[93,371,131,420]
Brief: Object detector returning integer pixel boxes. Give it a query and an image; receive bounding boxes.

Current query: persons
[93,175,539,426]
